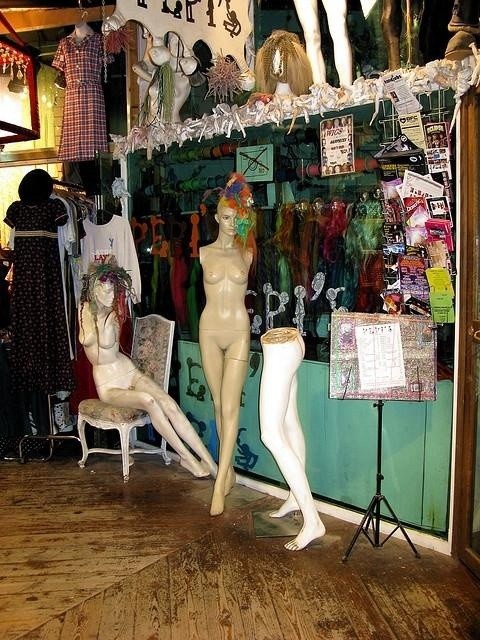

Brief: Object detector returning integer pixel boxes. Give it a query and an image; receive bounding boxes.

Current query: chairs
[76,314,179,485]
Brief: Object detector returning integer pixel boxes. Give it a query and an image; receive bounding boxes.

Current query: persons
[199,172,254,516]
[149,76,190,124]
[132,60,159,112]
[259,325,327,552]
[320,115,354,176]
[78,257,218,480]
[255,29,313,101]
[51,19,108,163]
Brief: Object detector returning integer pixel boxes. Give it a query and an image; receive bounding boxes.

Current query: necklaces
[293,0,353,94]
